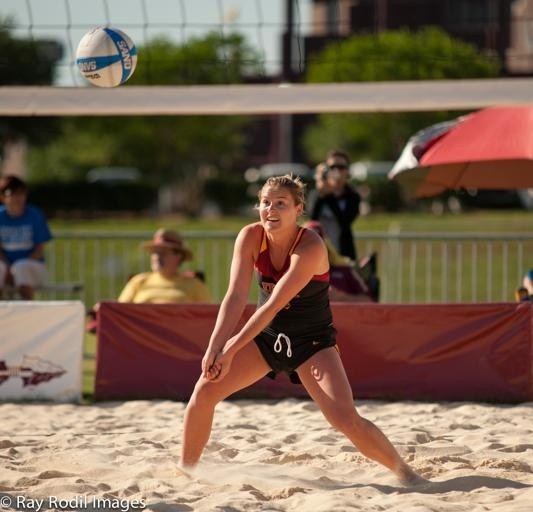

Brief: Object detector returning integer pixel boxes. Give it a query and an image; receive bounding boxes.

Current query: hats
[141,227,194,261]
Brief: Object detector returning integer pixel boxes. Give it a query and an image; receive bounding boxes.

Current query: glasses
[330,164,346,170]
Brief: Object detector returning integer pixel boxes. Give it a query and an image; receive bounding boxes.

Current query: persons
[179,175,408,473]
[119,229,215,304]
[0,175,52,302]
[307,152,362,263]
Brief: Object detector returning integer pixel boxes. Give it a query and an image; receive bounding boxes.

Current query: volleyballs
[76,26,138,88]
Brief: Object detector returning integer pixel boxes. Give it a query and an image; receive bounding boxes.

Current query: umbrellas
[419,106,533,193]
